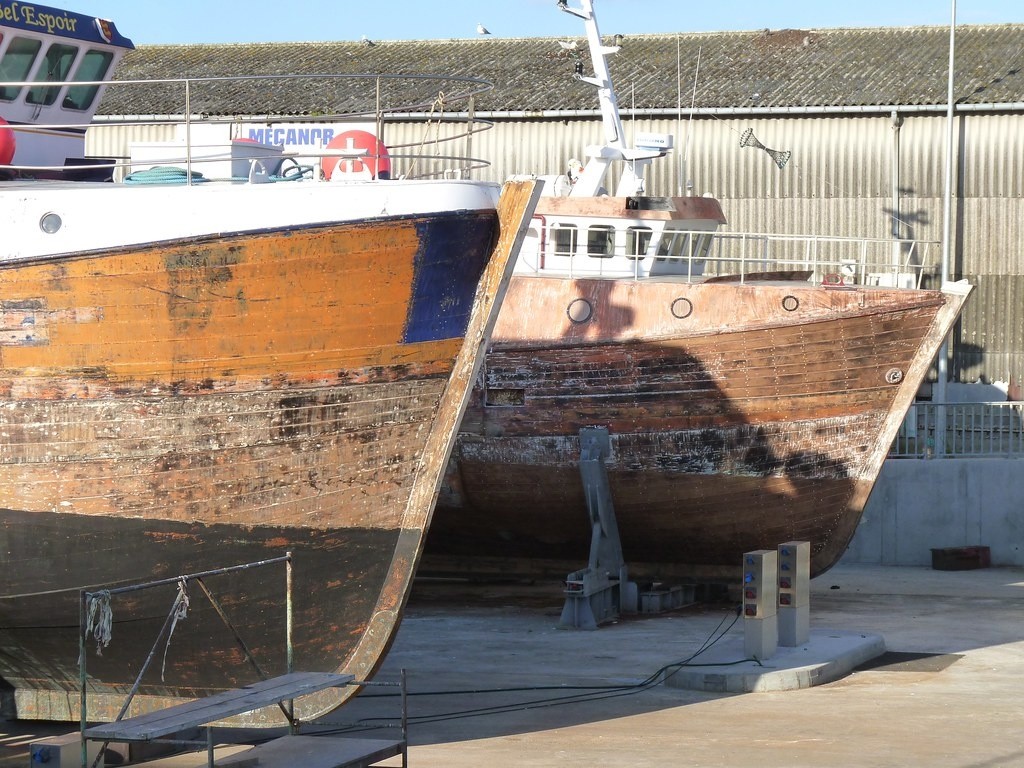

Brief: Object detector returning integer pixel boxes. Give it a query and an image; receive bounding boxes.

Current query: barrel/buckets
[232,142,284,183]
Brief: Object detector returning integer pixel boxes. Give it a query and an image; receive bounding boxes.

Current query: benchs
[79,552,408,768]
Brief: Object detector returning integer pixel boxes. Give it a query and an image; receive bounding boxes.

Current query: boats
[422,195,975,590]
[0,0,545,735]
